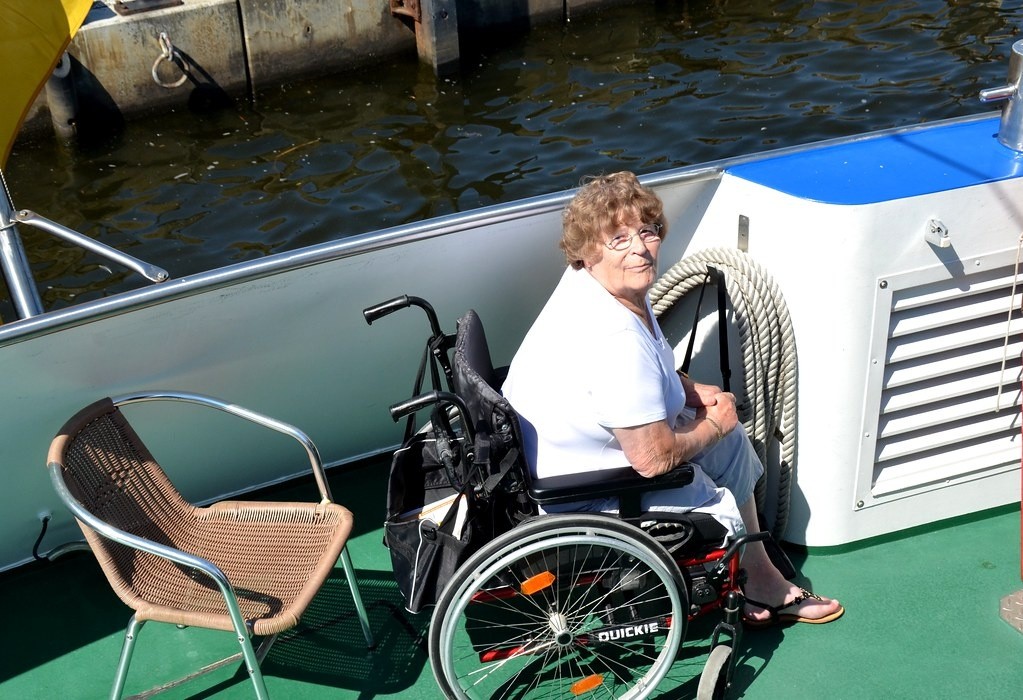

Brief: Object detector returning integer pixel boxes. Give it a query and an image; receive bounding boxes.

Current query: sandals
[743,587,846,627]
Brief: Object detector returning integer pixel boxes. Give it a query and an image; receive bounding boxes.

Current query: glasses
[602,224,663,251]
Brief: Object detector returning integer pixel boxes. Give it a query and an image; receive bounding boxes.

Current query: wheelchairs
[363,292,767,700]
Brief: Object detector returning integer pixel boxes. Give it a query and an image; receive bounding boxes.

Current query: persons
[500,168,845,626]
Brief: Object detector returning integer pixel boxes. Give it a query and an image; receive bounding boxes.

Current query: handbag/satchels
[381,332,481,614]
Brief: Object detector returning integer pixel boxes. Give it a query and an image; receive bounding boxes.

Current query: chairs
[44,389,376,700]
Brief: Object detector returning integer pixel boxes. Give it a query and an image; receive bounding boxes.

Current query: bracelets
[694,416,724,441]
